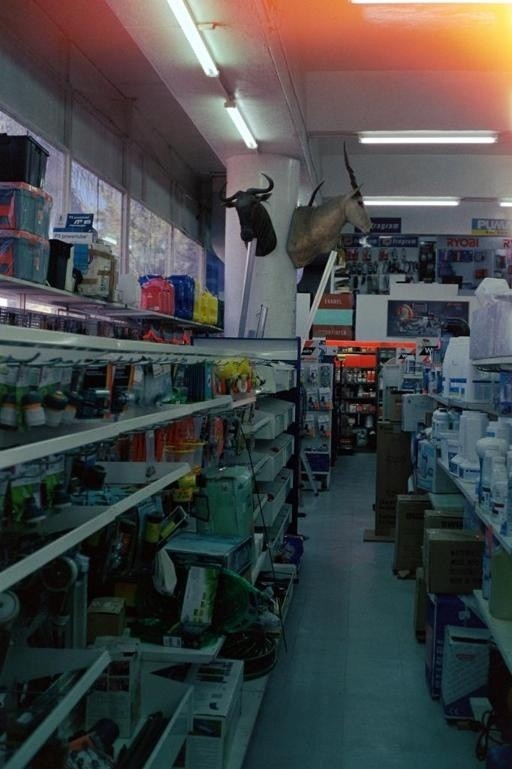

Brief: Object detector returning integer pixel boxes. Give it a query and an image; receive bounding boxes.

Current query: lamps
[360,195,465,208]
[356,129,501,145]
[164,0,226,79]
[222,97,261,153]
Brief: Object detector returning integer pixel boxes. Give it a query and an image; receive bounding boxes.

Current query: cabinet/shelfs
[334,352,380,454]
[432,354,510,676]
[0,320,313,768]
[422,391,491,521]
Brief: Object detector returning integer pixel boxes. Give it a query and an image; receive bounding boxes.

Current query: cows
[218,172,278,257]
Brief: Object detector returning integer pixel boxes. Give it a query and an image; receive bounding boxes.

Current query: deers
[285,140,375,269]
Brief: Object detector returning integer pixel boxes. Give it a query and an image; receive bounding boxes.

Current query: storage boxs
[0,230,53,285]
[0,180,56,242]
[1,131,53,191]
[371,415,495,724]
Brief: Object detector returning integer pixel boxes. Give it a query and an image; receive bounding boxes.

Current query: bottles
[335,370,375,383]
[489,455,507,524]
